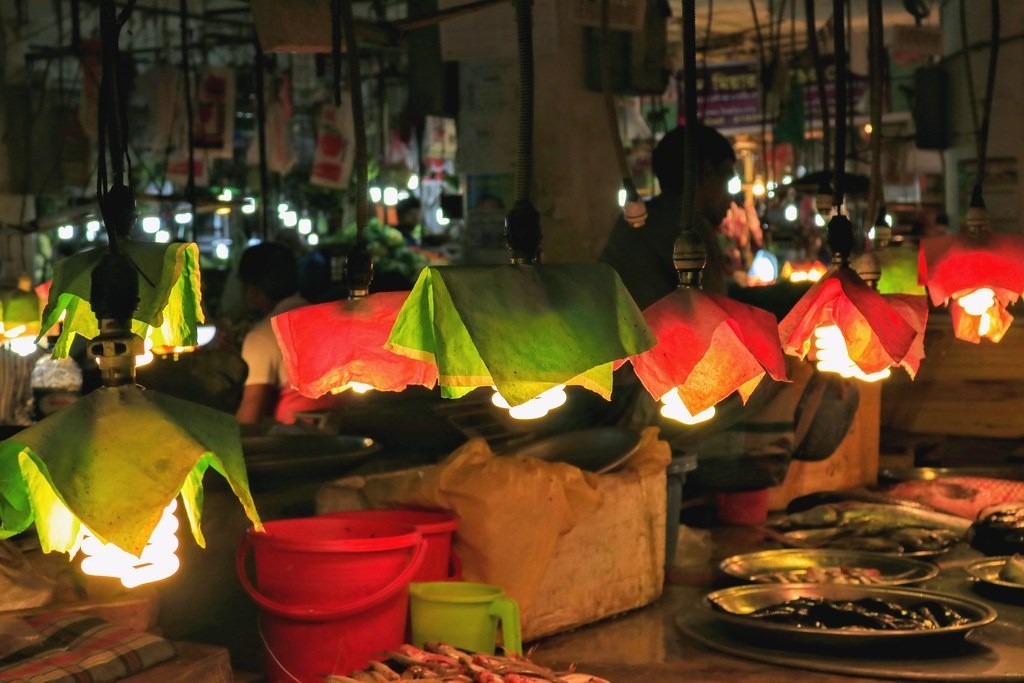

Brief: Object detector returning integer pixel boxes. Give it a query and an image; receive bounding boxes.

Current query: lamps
[1,0,1024,588]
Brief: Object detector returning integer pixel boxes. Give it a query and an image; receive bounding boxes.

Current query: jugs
[409,581,522,659]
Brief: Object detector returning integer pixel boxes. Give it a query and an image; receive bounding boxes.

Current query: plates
[502,424,646,475]
[694,465,1024,642]
[212,433,382,488]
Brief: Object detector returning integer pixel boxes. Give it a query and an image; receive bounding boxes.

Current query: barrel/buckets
[236,508,464,683]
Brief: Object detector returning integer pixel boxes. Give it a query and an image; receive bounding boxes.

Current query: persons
[394,199,421,247]
[324,207,344,237]
[576,122,737,427]
[236,241,346,425]
[936,215,955,235]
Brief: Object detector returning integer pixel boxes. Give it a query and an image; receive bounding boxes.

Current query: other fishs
[764,475,1024,556]
[317,638,610,682]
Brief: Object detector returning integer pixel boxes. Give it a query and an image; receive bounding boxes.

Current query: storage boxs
[767,376,888,516]
[315,442,667,648]
